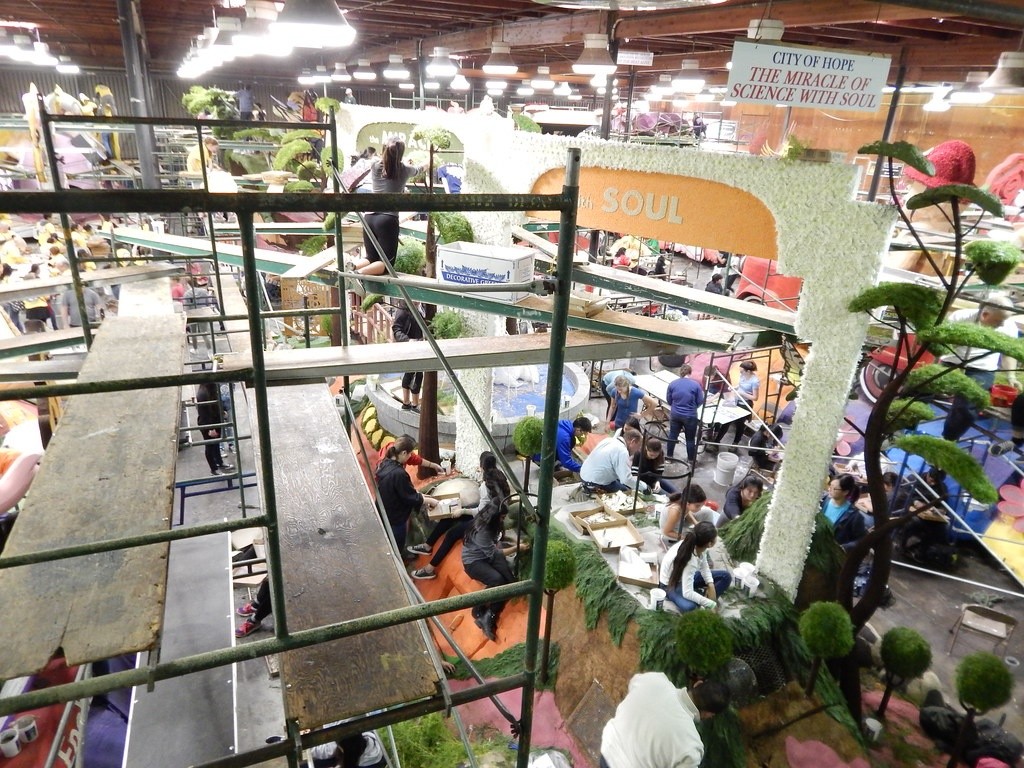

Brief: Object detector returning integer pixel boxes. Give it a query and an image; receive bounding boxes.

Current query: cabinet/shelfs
[1,95,583,768]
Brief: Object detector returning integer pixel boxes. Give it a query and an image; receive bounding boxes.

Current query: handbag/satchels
[11,299,25,313]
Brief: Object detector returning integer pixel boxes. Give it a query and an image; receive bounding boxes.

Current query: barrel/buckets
[715,452,739,487]
[0,728,22,758]
[13,714,39,744]
[991,384,1019,408]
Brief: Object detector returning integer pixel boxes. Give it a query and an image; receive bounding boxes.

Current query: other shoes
[401,402,412,411]
[218,463,234,472]
[411,407,421,416]
[346,271,367,298]
[265,735,285,745]
[687,460,697,467]
[728,441,739,453]
[706,441,719,453]
[344,263,357,289]
[666,456,673,464]
[209,469,226,476]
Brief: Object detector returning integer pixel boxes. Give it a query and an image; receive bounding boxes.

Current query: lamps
[924,52,1024,111]
[0,24,79,73]
[177,0,784,109]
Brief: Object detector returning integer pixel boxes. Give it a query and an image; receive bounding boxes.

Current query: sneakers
[1015,454,1024,464]
[407,544,433,555]
[411,566,436,580]
[989,440,1017,457]
[235,619,262,638]
[236,603,256,617]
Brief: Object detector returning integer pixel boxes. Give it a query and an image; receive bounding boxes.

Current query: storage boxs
[426,493,461,520]
[437,241,536,305]
[570,489,661,589]
[567,289,609,317]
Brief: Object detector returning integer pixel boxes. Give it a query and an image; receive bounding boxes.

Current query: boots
[475,610,496,642]
[472,602,487,619]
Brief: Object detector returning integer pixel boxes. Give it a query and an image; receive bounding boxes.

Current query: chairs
[948,602,1019,663]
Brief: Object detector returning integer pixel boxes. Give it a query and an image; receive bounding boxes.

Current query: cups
[650,588,666,611]
[527,405,536,415]
[639,552,658,566]
[733,567,745,589]
[744,577,759,598]
[565,395,571,408]
[865,718,882,743]
[740,562,755,576]
[655,504,664,521]
[1005,655,1020,675]
[441,461,452,476]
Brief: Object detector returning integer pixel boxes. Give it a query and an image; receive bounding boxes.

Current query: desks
[637,370,751,453]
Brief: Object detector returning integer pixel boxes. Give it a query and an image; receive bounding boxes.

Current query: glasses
[831,487,841,492]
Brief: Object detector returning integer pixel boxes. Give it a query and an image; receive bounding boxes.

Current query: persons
[990,392,1024,457]
[599,230,745,317]
[334,733,386,768]
[236,575,272,638]
[600,672,730,768]
[0,213,152,329]
[187,138,220,172]
[393,299,436,413]
[196,380,237,476]
[344,88,356,105]
[344,135,435,296]
[533,350,793,614]
[373,432,531,675]
[881,297,1022,448]
[812,450,955,595]
[186,84,267,144]
[172,261,214,359]
